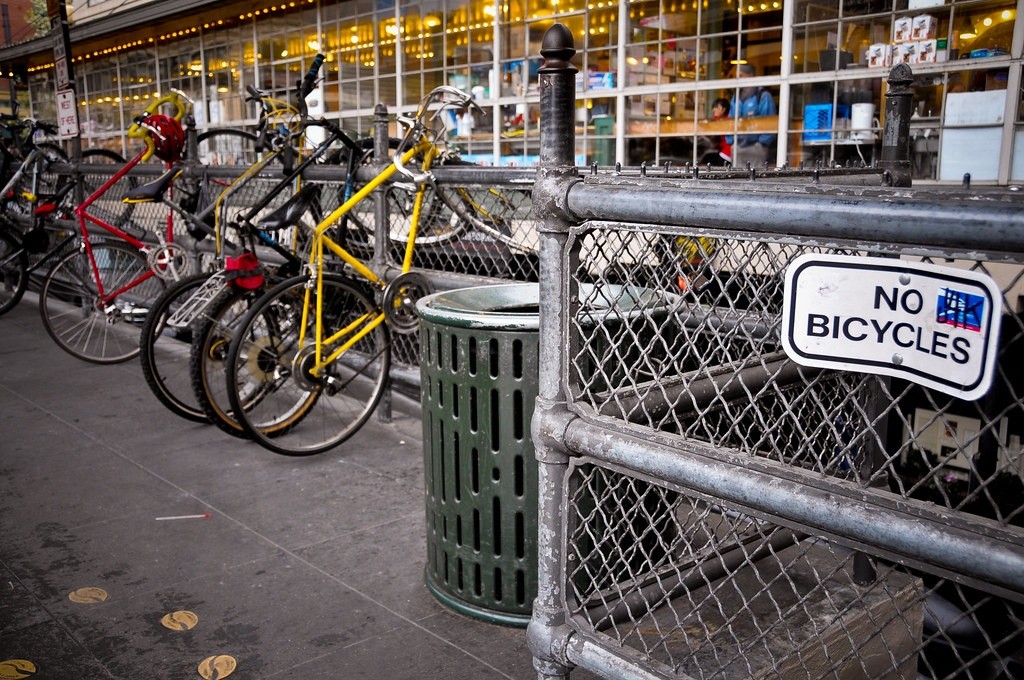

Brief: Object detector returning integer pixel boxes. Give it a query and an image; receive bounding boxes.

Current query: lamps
[959,15,977,41]
[730,44,748,65]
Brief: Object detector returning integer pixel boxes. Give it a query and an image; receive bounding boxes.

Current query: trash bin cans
[415,282,687,630]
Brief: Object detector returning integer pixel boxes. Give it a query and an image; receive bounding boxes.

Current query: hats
[739,62,754,72]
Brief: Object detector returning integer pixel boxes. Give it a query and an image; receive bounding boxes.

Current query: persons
[591,98,609,115]
[711,98,731,166]
[726,64,775,168]
[455,110,475,136]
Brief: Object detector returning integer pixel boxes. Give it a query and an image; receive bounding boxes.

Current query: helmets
[143,114,185,162]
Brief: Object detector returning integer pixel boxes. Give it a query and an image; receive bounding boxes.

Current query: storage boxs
[804,102,851,140]
[867,13,948,68]
[571,67,629,92]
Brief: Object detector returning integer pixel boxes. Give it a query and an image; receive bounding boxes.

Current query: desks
[799,138,877,168]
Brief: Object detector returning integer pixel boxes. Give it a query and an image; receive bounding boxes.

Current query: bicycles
[0,52,517,457]
